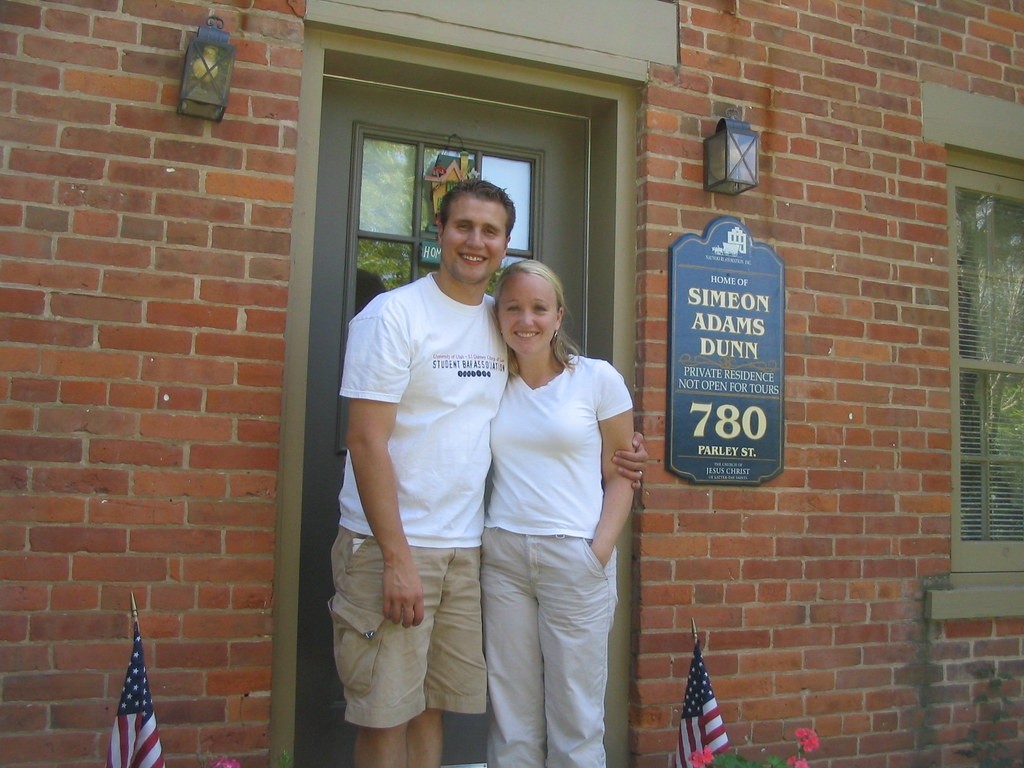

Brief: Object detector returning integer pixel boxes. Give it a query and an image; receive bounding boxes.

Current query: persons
[479,259,634,768]
[328,180,650,765]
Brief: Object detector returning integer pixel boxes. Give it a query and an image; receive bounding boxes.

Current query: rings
[639,470,643,477]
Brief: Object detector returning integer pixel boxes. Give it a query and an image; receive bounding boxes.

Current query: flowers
[208,754,240,768]
[686,727,818,767]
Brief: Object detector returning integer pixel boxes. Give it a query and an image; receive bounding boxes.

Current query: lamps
[176,16,237,124]
[702,108,759,196]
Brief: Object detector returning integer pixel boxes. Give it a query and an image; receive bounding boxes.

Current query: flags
[105,594,166,768]
[676,617,729,768]
